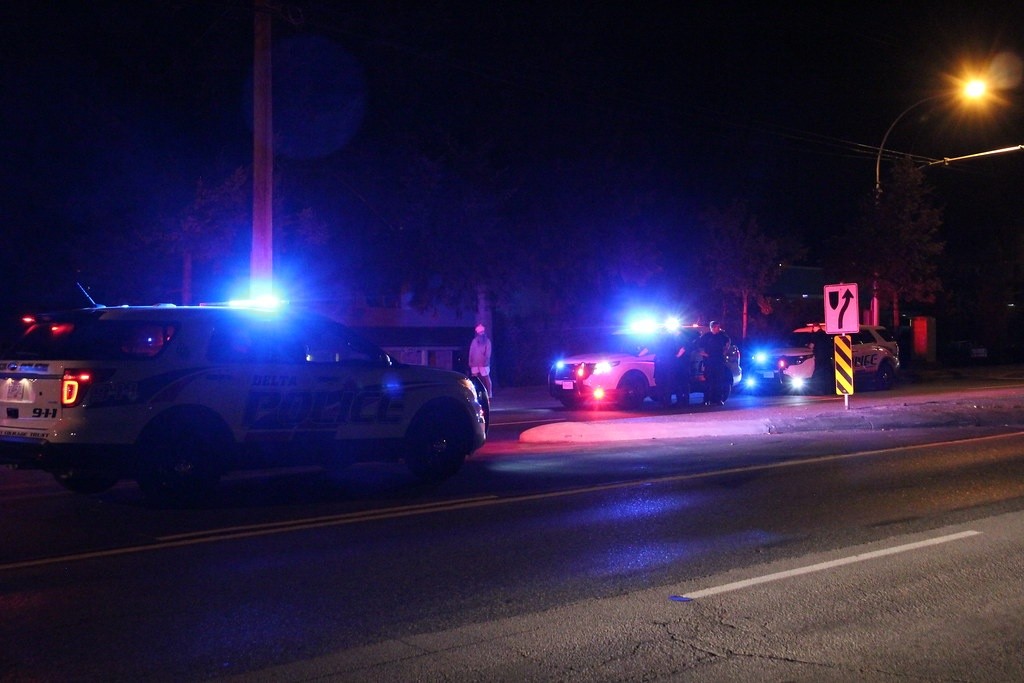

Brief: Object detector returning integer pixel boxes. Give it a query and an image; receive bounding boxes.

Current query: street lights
[875,71,997,322]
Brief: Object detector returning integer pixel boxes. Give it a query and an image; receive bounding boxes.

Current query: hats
[475,324,485,333]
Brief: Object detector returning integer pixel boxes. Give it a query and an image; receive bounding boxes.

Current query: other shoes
[720,400,724,406]
[705,401,711,406]
[671,403,687,409]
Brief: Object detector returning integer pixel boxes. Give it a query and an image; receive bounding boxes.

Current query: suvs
[546,325,742,411]
[0,304,487,507]
[751,323,901,390]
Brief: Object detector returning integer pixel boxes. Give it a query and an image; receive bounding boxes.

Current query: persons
[638,324,690,408]
[468,325,492,399]
[693,320,731,406]
[809,323,831,396]
